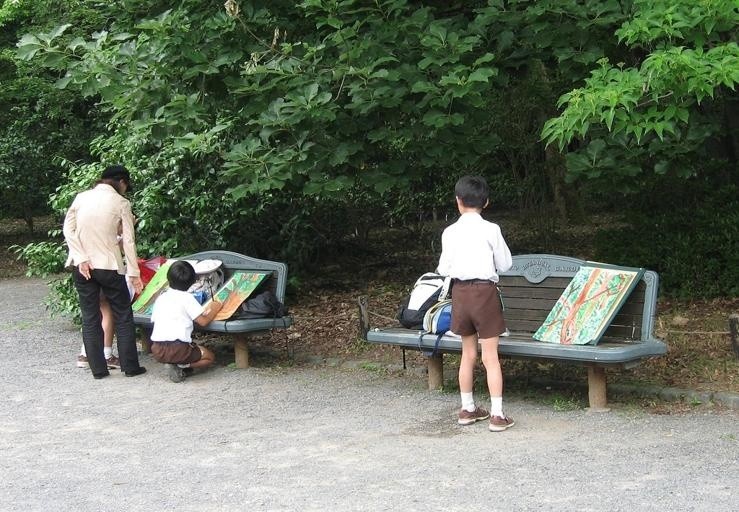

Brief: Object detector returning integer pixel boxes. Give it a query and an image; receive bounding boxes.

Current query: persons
[436,177,514,432]
[62,165,147,378]
[150,261,214,383]
[76,212,136,369]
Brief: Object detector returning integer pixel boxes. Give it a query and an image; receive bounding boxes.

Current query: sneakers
[458,408,489,426]
[489,416,514,432]
[77,355,121,379]
[169,363,186,383]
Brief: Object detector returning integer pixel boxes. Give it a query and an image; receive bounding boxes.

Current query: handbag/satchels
[238,291,283,319]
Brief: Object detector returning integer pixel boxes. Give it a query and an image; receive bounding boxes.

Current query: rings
[86,270,90,273]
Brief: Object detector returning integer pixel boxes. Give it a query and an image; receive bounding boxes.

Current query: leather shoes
[126,367,146,377]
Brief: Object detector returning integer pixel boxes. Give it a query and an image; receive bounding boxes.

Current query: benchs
[363,250,667,415]
[109,248,295,369]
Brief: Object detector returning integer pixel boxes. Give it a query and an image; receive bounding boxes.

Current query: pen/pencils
[209,286,214,302]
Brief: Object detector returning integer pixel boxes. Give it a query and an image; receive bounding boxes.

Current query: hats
[102,165,132,191]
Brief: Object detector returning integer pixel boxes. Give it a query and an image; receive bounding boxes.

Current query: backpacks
[396,272,453,335]
